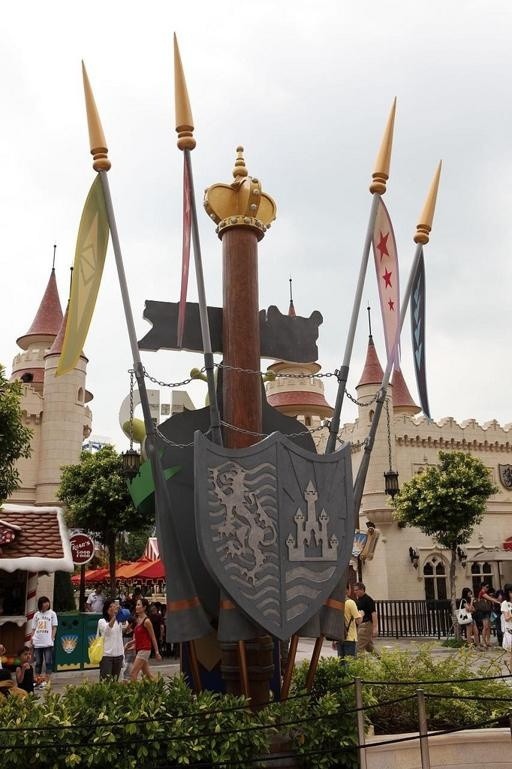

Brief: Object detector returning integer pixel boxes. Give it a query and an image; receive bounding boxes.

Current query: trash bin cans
[55,611,103,672]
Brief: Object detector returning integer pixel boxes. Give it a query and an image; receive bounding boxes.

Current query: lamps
[408,546,420,571]
[120,370,142,485]
[381,400,401,500]
[457,546,468,569]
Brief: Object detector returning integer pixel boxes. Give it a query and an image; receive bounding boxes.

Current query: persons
[0,596,58,700]
[83,583,179,683]
[457,582,512,673]
[332,581,365,658]
[351,584,382,659]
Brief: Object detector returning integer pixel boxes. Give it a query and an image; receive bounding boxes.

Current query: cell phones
[114,600,119,606]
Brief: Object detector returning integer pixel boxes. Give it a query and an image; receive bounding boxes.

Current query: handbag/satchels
[475,599,494,612]
[455,607,473,625]
[87,635,106,665]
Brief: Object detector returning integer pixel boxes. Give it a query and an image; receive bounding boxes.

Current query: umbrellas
[71,554,166,584]
